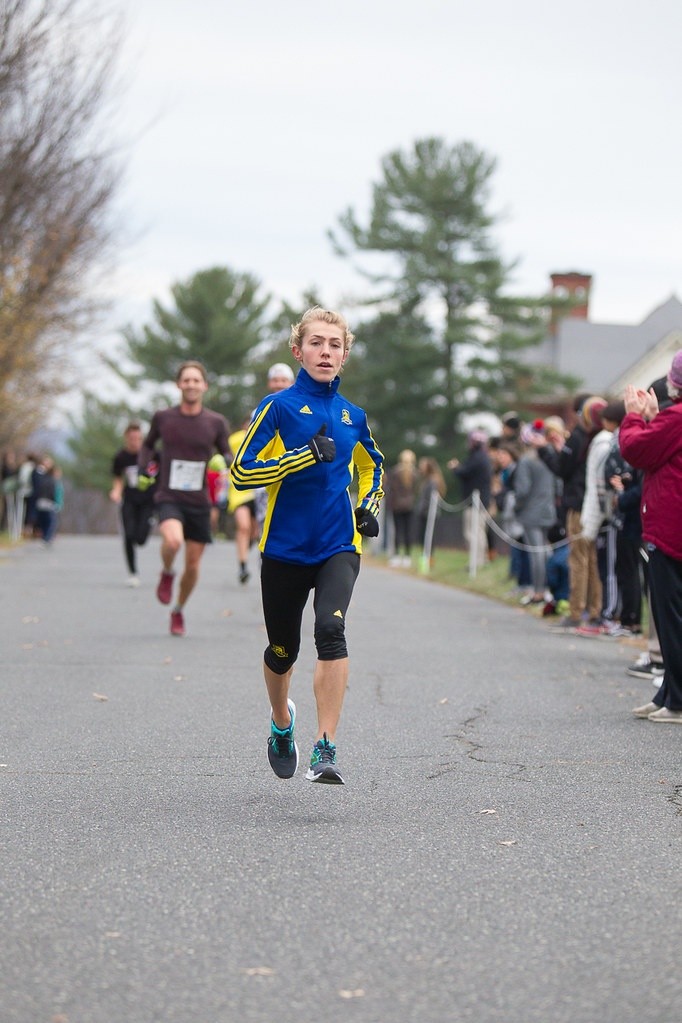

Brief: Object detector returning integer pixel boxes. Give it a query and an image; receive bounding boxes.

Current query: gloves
[309,423,335,463]
[355,507,379,537]
[208,453,226,471]
[138,475,156,490]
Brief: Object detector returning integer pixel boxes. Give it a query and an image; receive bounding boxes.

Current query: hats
[596,400,625,421]
[521,419,547,447]
[668,352,682,386]
[268,362,294,383]
[582,397,608,428]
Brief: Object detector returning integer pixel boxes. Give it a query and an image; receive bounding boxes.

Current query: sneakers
[306,732,345,784]
[267,698,299,779]
[521,590,682,722]
[157,571,174,604]
[171,610,183,634]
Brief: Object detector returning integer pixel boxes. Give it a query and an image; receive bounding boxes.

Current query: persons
[230,308,385,784]
[387,450,421,568]
[249,362,296,534]
[206,451,228,542]
[618,350,682,722]
[109,423,157,588]
[135,360,230,636]
[418,456,445,569]
[448,376,671,687]
[228,414,264,582]
[0,448,63,543]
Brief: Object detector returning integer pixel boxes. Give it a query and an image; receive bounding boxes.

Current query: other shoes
[240,569,248,583]
